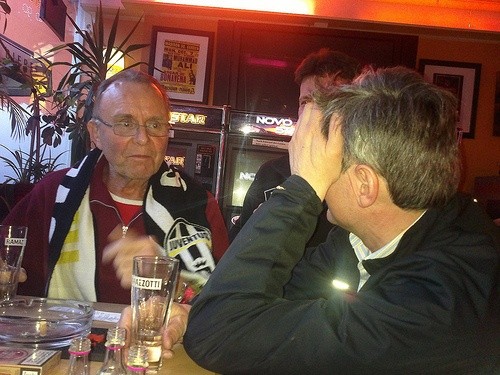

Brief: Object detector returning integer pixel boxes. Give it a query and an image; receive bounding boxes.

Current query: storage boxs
[0,347,62,375]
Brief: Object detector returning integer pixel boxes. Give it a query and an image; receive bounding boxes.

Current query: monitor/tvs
[223,143,286,210]
[212,17,418,121]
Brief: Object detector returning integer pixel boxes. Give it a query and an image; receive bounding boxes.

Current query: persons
[0,69,230,305]
[227,51,359,248]
[119,67,500,375]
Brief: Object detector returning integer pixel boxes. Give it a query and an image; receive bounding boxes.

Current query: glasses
[92,116,173,137]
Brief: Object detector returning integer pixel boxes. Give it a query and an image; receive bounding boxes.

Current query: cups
[0,225,28,306]
[131,256,180,374]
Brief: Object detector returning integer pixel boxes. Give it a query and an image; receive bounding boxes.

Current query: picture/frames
[148,26,215,105]
[419,58,481,139]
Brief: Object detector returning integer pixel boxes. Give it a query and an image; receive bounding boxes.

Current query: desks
[0,295,216,375]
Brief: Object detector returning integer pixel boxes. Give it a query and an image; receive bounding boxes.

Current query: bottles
[127,345,148,375]
[68,338,91,375]
[96,327,127,375]
